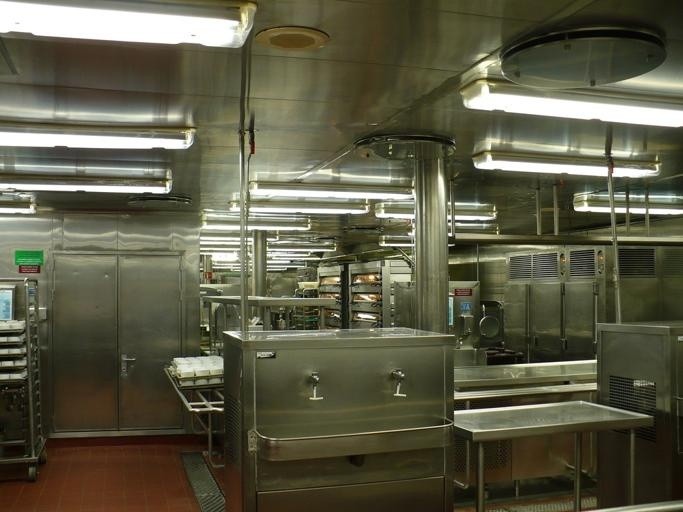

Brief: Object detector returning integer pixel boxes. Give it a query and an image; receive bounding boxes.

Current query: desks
[452,399,653,512]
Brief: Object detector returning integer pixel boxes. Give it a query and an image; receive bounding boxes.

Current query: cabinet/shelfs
[293,287,319,329]
[0,277,46,481]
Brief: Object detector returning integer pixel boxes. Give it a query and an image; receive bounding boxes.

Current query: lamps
[0,0,683,271]
